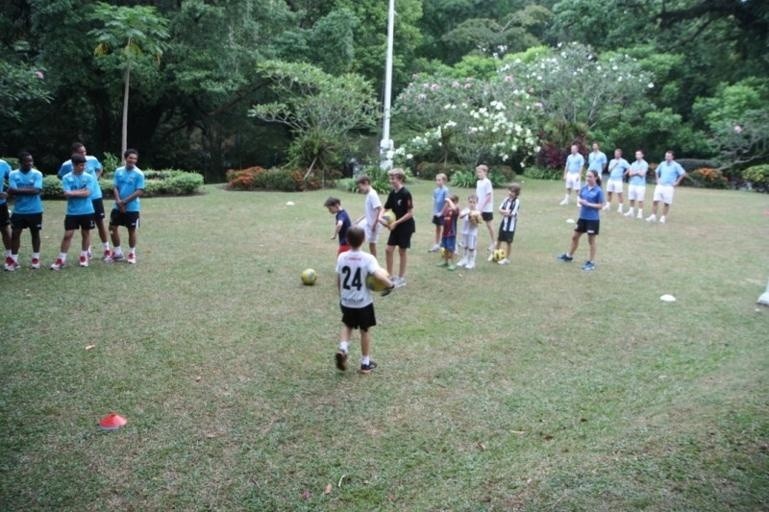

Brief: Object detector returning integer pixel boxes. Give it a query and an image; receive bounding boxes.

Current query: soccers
[492,248,506,262]
[366,268,392,291]
[440,247,452,258]
[300,268,318,284]
[469,210,481,224]
[383,211,396,225]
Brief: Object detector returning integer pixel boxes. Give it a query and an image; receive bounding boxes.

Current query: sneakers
[2,250,21,271]
[579,262,595,270]
[102,248,136,264]
[334,349,348,371]
[360,360,377,373]
[487,242,511,266]
[387,274,407,289]
[556,253,573,262]
[49,257,65,272]
[427,245,476,272]
[79,246,93,266]
[30,252,41,271]
[559,197,667,225]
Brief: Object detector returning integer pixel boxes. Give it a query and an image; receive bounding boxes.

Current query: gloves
[380,285,395,297]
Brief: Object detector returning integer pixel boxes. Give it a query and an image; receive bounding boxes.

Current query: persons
[587,141,607,179]
[646,150,686,223]
[324,168,415,288]
[603,148,630,213]
[560,143,584,207]
[335,227,394,374]
[558,169,604,271]
[624,149,648,218]
[0,142,144,269]
[431,165,521,270]
